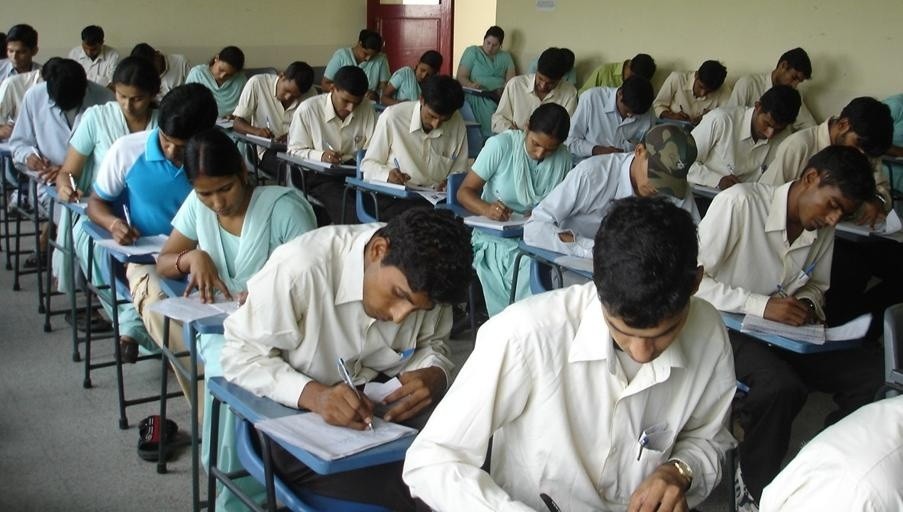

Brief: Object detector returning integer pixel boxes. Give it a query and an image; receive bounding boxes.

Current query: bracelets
[175,249,190,275]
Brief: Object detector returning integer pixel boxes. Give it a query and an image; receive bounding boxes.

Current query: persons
[7,58,117,228]
[287,65,376,223]
[0,68,43,140]
[184,45,246,125]
[758,394,903,512]
[380,47,443,105]
[577,54,657,98]
[522,124,702,290]
[758,97,894,241]
[690,145,882,504]
[563,75,656,159]
[319,29,390,98]
[457,26,516,133]
[401,195,738,512]
[67,24,119,88]
[231,61,318,189]
[687,86,802,190]
[491,47,577,134]
[653,60,731,123]
[157,131,317,512]
[220,208,474,511]
[131,42,191,100]
[456,102,573,318]
[359,74,469,221]
[727,47,819,131]
[0,23,43,86]
[87,83,219,422]
[56,57,161,363]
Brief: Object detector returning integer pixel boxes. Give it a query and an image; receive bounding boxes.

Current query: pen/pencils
[679,104,685,111]
[495,189,512,225]
[637,437,646,460]
[68,170,82,206]
[776,284,788,299]
[121,203,138,245]
[338,357,375,434]
[325,142,345,165]
[32,138,44,166]
[393,157,409,186]
[726,163,739,178]
[539,492,560,512]
[799,261,816,280]
[266,115,270,129]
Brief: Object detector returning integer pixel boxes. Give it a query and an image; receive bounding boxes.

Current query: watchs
[875,192,887,207]
[664,460,693,483]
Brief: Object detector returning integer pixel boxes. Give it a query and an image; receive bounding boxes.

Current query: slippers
[23,241,48,268]
[137,415,173,460]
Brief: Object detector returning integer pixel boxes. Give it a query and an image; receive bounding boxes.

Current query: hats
[644,122,699,201]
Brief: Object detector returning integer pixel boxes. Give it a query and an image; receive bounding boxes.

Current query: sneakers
[112,338,139,364]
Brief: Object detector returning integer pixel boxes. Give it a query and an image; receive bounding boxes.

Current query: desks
[836,214,903,251]
[232,130,287,185]
[155,265,228,509]
[517,239,593,295]
[346,149,417,223]
[42,185,91,362]
[716,306,861,510]
[275,152,353,224]
[443,172,523,329]
[691,182,723,200]
[77,218,150,428]
[204,375,416,512]
